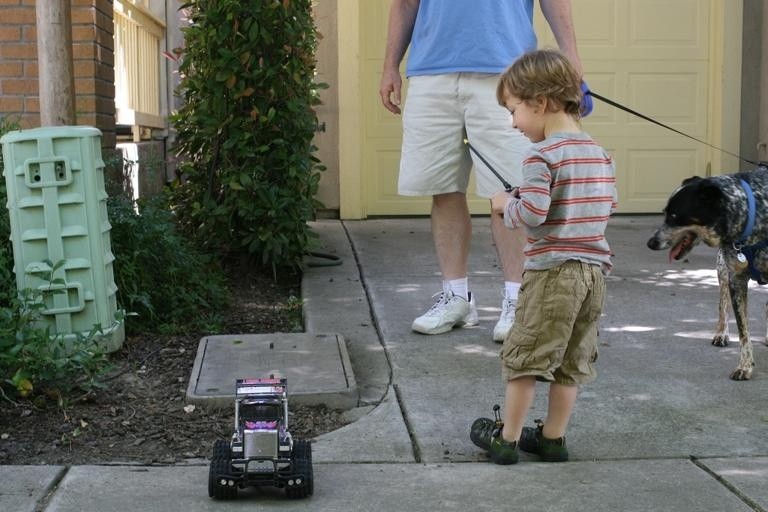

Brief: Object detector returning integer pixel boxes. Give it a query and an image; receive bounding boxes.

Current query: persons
[467,50,622,467]
[379,0,584,344]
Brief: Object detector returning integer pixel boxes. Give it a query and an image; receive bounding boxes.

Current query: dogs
[647,163,768,380]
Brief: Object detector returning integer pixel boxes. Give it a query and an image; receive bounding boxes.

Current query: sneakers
[470,416,520,466]
[411,292,480,336]
[520,424,568,462]
[490,295,521,343]
